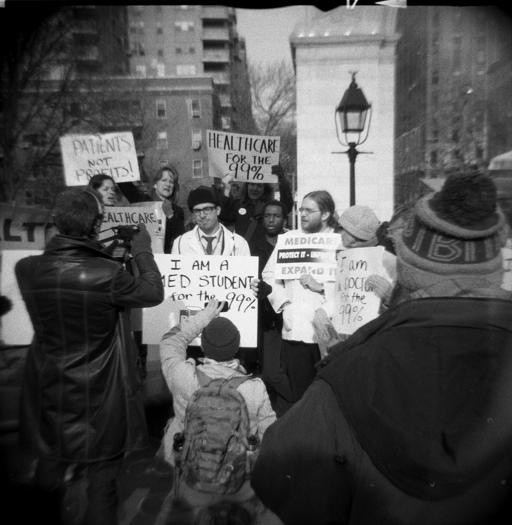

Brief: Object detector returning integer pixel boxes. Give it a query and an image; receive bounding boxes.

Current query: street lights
[326,66,378,208]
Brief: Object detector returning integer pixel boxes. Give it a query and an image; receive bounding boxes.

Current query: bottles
[172,431,185,468]
[243,435,259,474]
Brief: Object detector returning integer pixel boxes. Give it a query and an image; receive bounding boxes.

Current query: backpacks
[174,369,261,495]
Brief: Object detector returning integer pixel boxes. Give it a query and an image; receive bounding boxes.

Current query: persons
[247,169,512,525]
[13,187,166,524]
[156,295,287,524]
[89,163,395,413]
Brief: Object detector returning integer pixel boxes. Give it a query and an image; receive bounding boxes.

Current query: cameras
[205,301,228,313]
[117,224,137,241]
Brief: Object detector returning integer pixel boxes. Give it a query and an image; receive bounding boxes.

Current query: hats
[338,205,379,241]
[188,185,218,212]
[201,318,240,360]
[397,172,504,289]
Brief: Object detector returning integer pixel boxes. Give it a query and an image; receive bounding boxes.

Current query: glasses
[193,207,215,215]
